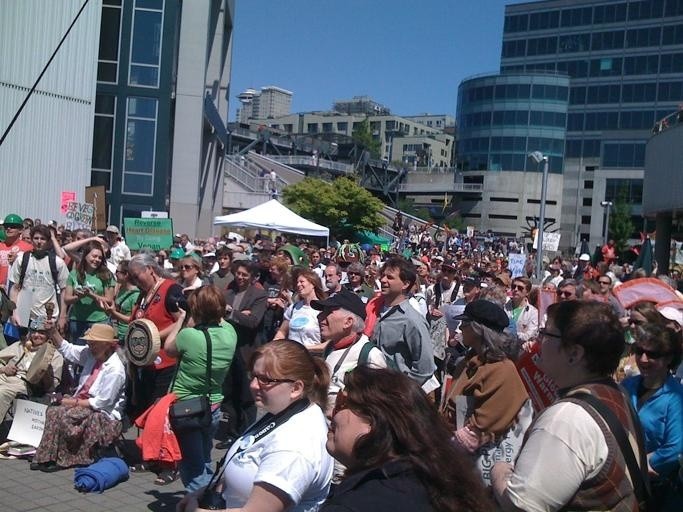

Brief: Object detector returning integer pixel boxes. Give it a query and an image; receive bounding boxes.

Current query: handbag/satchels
[170,325,212,435]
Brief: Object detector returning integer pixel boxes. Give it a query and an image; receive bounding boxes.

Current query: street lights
[600,201,612,244]
[529,150,549,281]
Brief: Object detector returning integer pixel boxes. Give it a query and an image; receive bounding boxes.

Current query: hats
[310,292,367,322]
[30,315,46,331]
[79,323,113,342]
[462,275,481,286]
[453,301,510,330]
[440,260,458,271]
[579,253,590,260]
[106,225,118,233]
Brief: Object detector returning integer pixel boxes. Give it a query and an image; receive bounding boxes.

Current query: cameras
[166,291,192,313]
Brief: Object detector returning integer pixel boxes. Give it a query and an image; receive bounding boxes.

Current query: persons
[501,277,538,344]
[127,255,195,485]
[97,260,140,346]
[30,320,126,474]
[271,271,331,354]
[259,168,277,194]
[610,302,657,383]
[175,339,335,511]
[0,320,63,427]
[8,224,70,339]
[382,210,683,301]
[163,285,238,494]
[364,260,438,388]
[320,366,490,511]
[152,229,215,285]
[215,237,381,291]
[441,300,533,486]
[21,214,130,269]
[64,241,116,345]
[309,292,387,431]
[620,323,683,512]
[490,300,651,512]
[0,214,32,298]
[659,305,683,333]
[425,260,465,361]
[214,262,268,450]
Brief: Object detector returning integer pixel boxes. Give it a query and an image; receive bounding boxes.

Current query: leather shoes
[30,463,42,470]
[215,435,234,449]
[41,464,58,473]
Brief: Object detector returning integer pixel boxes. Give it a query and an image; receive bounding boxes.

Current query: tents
[211,199,330,249]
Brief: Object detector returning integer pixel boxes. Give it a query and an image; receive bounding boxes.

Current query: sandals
[155,467,180,485]
[131,459,157,472]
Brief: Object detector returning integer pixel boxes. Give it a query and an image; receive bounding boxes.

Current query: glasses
[441,268,452,273]
[556,290,572,297]
[247,370,295,385]
[511,284,524,290]
[598,281,609,284]
[632,345,663,360]
[349,272,359,275]
[180,264,194,270]
[537,328,562,340]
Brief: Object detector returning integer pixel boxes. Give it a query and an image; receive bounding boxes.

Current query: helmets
[4,214,23,227]
